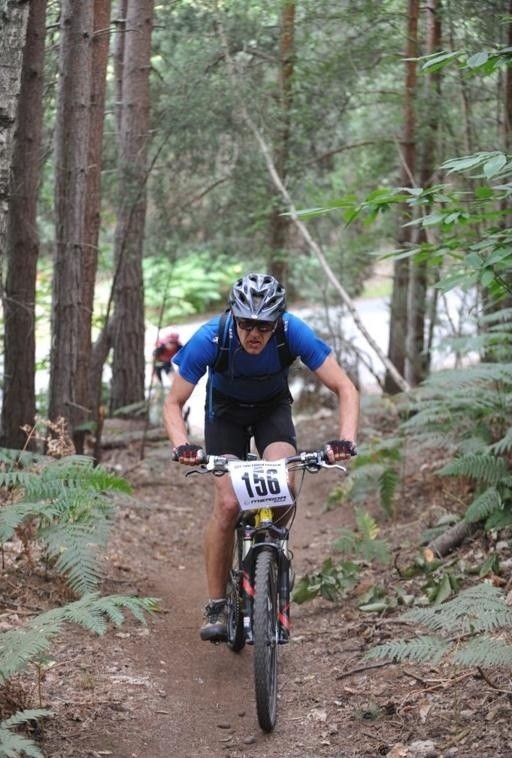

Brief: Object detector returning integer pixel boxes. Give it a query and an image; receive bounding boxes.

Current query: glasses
[236,317,277,332]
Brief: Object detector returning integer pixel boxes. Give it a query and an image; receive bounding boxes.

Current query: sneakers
[200,601,229,641]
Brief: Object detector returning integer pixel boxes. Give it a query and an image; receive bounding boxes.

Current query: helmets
[228,272,287,322]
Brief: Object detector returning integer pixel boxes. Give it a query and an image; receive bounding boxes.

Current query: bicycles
[172,440,359,737]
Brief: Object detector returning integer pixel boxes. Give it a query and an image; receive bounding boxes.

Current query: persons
[152,333,183,386]
[163,272,360,641]
